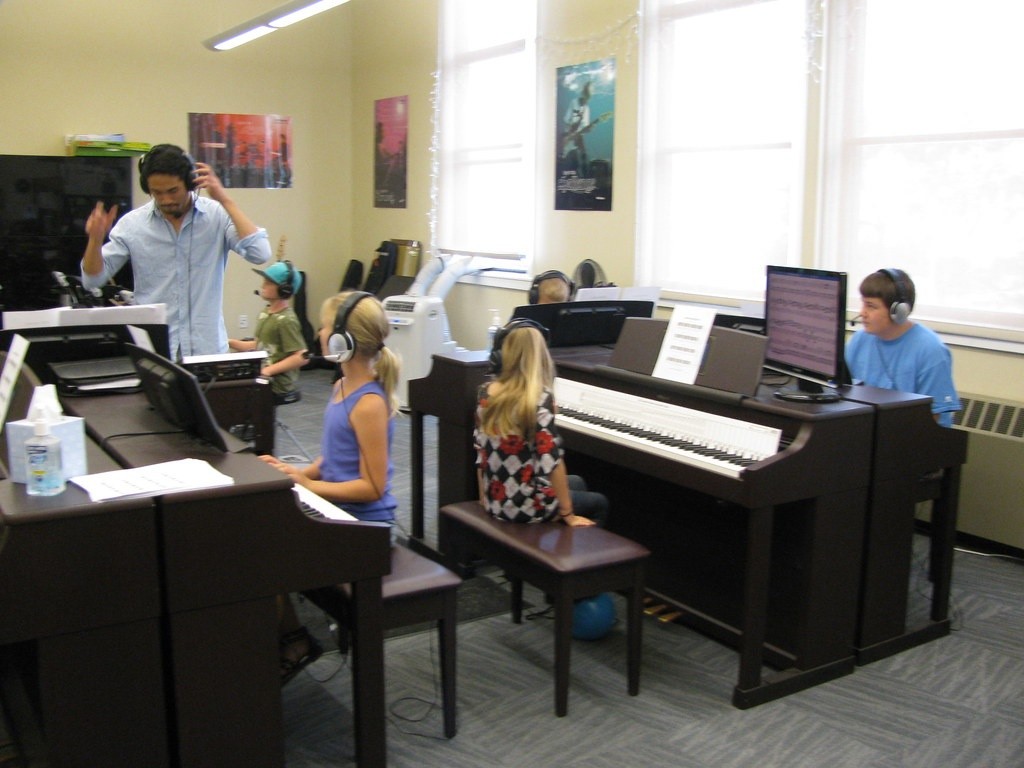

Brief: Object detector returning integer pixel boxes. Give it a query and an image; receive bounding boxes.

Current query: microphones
[850,315,862,327]
[303,351,340,362]
[255,290,287,299]
[174,188,200,218]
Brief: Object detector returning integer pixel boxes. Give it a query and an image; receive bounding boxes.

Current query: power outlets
[239,315,248,328]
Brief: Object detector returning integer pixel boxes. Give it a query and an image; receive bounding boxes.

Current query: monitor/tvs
[763,265,847,403]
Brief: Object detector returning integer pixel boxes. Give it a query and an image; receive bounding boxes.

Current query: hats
[252,261,301,293]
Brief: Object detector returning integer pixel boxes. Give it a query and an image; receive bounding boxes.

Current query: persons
[80,144,271,364]
[842,270,964,437]
[226,259,312,441]
[473,318,608,606]
[254,289,398,684]
[528,270,574,306]
[560,80,594,160]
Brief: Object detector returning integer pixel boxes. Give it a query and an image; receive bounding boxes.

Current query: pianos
[0,323,394,768]
[398,297,970,710]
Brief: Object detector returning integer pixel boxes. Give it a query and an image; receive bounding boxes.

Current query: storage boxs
[65,133,153,157]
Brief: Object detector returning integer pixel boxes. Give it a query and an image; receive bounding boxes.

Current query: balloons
[571,593,617,640]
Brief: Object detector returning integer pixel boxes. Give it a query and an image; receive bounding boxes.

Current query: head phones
[278,260,295,298]
[878,268,911,324]
[138,150,200,192]
[489,317,551,372]
[529,270,576,305]
[328,291,375,364]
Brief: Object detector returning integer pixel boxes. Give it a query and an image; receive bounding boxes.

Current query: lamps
[200,0,354,53]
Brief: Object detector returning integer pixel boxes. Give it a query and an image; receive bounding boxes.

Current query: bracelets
[559,510,572,517]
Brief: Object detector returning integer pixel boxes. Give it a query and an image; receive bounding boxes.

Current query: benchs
[296,542,464,739]
[439,495,654,719]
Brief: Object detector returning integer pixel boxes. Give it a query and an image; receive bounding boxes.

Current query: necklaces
[255,300,281,349]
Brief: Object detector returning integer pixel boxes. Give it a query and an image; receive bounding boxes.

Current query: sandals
[281,625,324,686]
